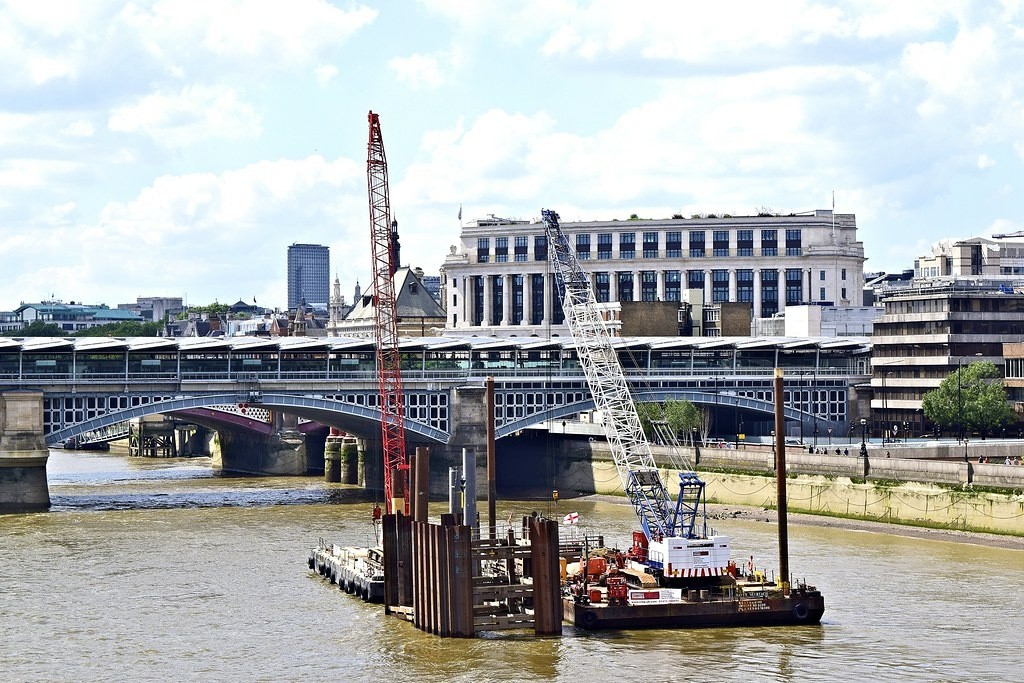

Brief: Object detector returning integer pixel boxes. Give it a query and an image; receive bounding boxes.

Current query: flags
[563,513,579,525]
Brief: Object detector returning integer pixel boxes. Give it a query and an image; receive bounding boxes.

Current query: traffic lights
[849,420,856,435]
[904,421,909,431]
[932,423,941,437]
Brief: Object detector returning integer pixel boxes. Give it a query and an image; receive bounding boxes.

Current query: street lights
[812,366,836,438]
[859,418,867,448]
[827,428,833,446]
[770,431,775,451]
[959,353,984,439]
[692,426,699,448]
[793,366,811,445]
[962,437,970,460]
[894,424,899,443]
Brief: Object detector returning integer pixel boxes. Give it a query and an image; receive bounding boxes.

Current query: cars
[786,438,810,446]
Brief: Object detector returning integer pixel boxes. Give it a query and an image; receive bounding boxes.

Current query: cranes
[364,107,413,524]
[533,205,729,593]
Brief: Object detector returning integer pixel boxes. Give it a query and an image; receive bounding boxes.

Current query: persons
[1013,457,1018,465]
[726,441,730,448]
[589,436,597,441]
[886,450,890,458]
[718,441,722,449]
[843,448,849,456]
[985,456,990,463]
[821,447,827,454]
[648,440,656,445]
[978,455,983,463]
[1004,457,1011,465]
[835,447,841,455]
[815,448,820,454]
[808,445,813,454]
